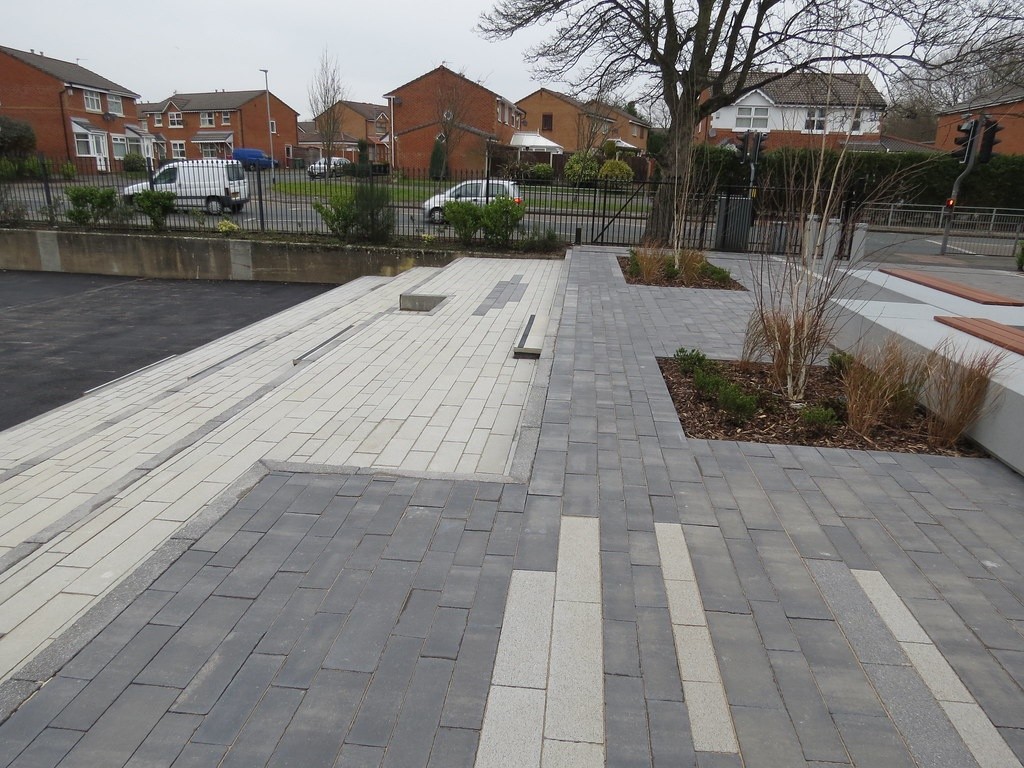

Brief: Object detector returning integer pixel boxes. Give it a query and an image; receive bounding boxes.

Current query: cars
[423,179,526,227]
[308,158,350,179]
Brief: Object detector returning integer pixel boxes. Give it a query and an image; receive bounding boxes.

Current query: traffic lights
[735,129,750,164]
[946,198,955,207]
[756,132,769,162]
[977,120,1004,163]
[954,119,975,164]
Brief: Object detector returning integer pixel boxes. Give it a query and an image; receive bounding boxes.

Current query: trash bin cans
[288,158,294,169]
[714,195,754,252]
[768,221,789,254]
[805,214,868,261]
[294,157,305,169]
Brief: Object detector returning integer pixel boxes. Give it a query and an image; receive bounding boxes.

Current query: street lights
[259,68,276,184]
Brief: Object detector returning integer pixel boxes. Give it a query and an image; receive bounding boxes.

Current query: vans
[123,160,251,216]
[232,149,279,172]
